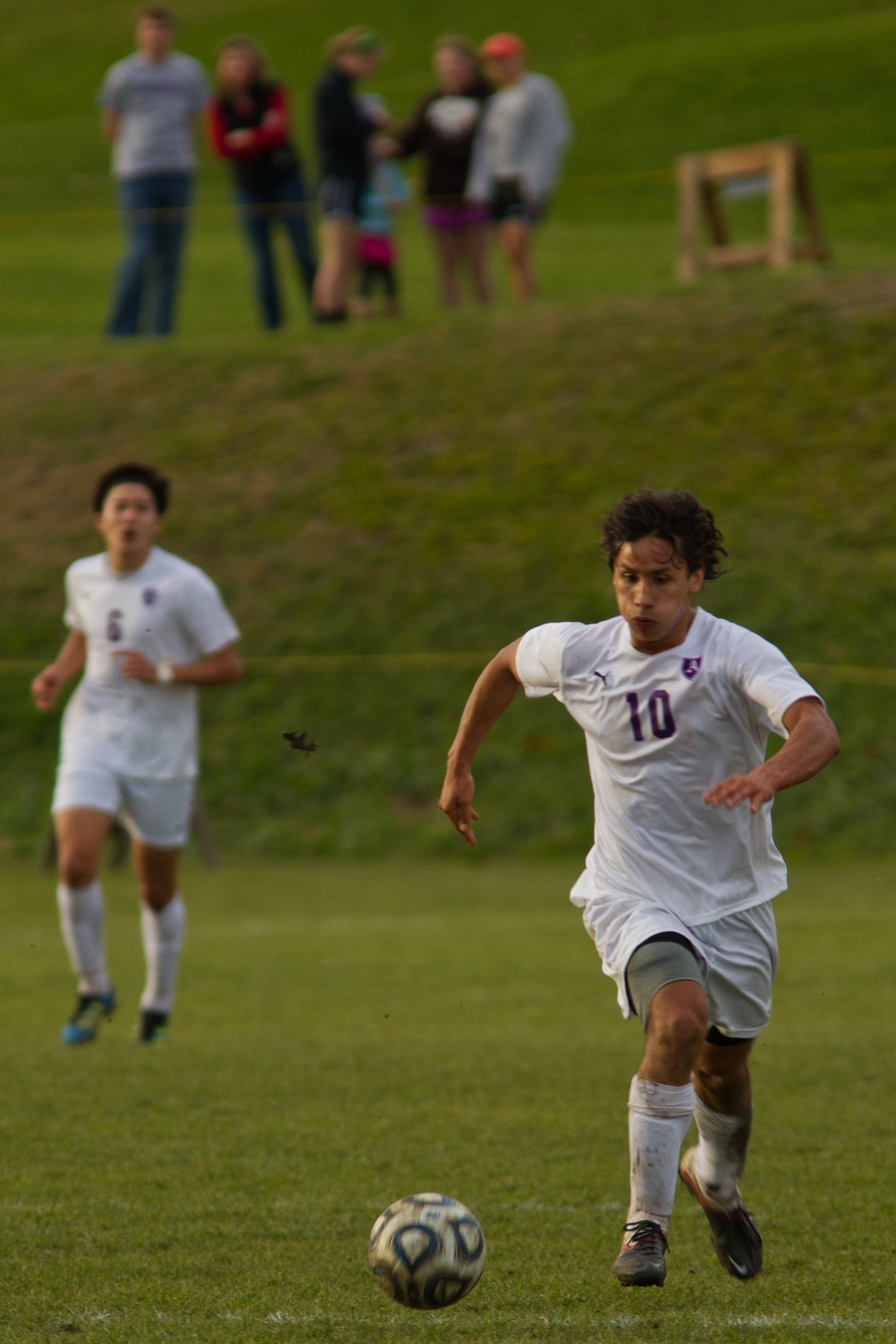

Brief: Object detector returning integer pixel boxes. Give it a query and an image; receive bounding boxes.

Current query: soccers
[369,1190,491,1311]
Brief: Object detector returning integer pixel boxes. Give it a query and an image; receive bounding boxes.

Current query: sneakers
[137,1009,171,1045]
[677,1144,764,1281]
[613,1219,670,1288]
[62,988,116,1046]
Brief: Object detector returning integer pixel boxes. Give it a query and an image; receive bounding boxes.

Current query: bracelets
[155,659,175,683]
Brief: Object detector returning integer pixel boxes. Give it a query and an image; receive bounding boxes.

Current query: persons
[202,37,320,331]
[300,22,389,322]
[92,7,209,339]
[353,131,409,321]
[376,39,492,310]
[439,492,853,1289]
[26,465,243,1049]
[459,33,573,308]
